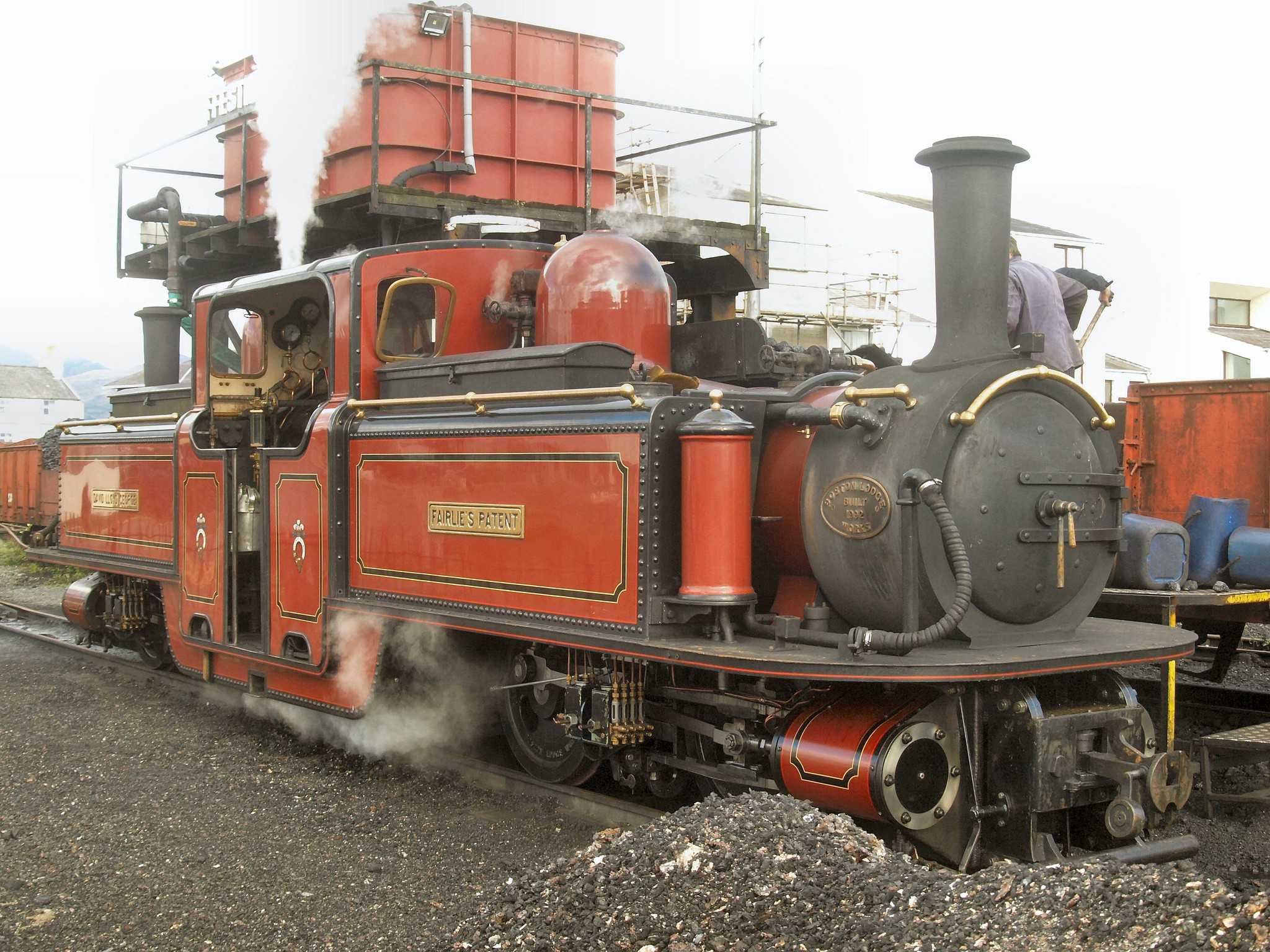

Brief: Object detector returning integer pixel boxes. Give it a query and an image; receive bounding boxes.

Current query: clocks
[271,296,321,352]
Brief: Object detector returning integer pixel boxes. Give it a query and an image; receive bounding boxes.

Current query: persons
[1006,236,1115,379]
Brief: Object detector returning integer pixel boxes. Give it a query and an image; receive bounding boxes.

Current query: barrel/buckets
[1104,510,1190,590]
[1184,492,1250,588]
[1228,526,1270,590]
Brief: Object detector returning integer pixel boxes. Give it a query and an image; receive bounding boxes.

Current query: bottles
[237,484,261,552]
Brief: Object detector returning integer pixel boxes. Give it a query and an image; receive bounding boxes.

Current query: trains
[0,1,1207,882]
